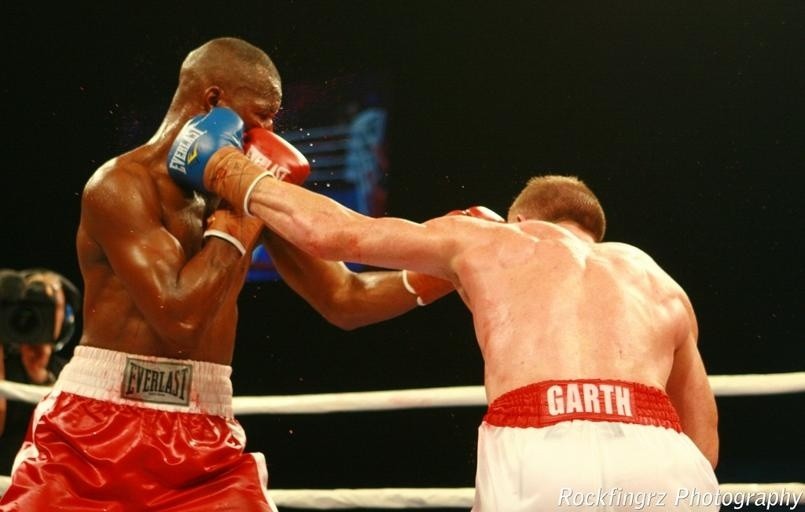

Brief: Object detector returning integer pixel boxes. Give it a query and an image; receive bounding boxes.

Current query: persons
[0,269,81,477]
[166,104,721,512]
[0,36,508,512]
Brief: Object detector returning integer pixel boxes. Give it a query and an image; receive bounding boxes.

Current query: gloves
[202,127,310,259]
[399,202,506,309]
[164,105,274,220]
